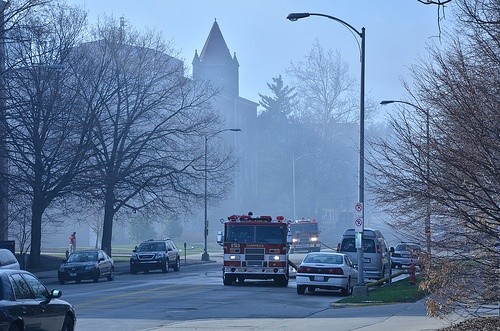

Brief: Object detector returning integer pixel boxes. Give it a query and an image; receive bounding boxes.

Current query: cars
[57,248,115,283]
[391,241,428,269]
[295,251,359,294]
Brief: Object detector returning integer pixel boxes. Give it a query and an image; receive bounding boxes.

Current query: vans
[337,228,395,284]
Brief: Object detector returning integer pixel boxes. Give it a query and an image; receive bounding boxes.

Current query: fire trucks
[216,212,291,288]
[200,129,242,259]
[290,218,322,253]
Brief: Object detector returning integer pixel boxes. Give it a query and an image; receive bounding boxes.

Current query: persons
[69,232,76,254]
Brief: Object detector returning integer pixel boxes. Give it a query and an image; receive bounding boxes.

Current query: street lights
[380,100,431,255]
[286,13,368,300]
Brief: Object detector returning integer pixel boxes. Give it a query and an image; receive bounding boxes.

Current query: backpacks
[68,236,74,244]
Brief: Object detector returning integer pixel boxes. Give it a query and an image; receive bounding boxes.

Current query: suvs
[0,269,76,331]
[0,249,20,270]
[129,239,180,273]
